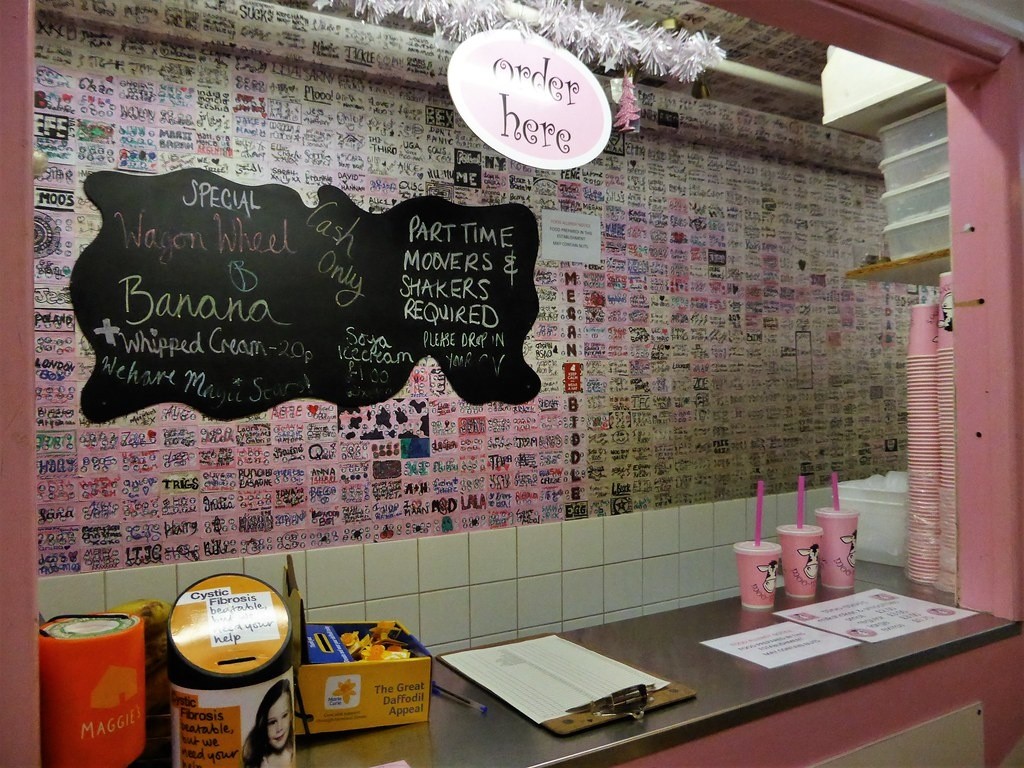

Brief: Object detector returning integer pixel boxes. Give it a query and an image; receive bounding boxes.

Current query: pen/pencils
[431,681,488,713]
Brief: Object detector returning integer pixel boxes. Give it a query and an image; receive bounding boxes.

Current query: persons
[242,679,294,768]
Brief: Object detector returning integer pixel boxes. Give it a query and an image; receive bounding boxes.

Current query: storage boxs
[280,554,434,739]
[877,101,952,258]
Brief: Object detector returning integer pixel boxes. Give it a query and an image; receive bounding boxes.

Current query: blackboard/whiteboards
[70,167,541,425]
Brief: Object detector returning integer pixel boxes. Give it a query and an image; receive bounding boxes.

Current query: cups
[776,524,824,598]
[733,541,782,609]
[815,507,860,588]
[905,270,959,594]
